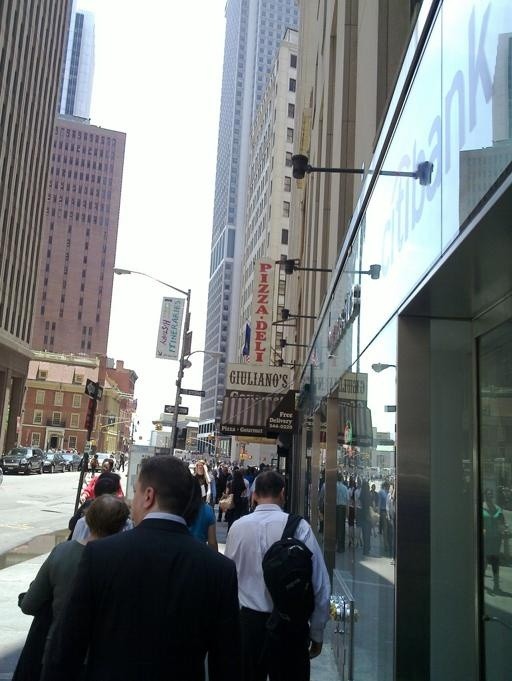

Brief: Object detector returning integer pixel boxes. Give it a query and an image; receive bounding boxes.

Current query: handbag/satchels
[219,494,234,510]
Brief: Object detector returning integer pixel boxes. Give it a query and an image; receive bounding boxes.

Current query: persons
[224,470,332,680]
[307,468,396,565]
[21,494,130,679]
[42,453,244,680]
[21,446,279,552]
[496,484,506,509]
[482,488,510,596]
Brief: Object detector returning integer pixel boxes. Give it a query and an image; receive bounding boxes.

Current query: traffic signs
[163,404,188,415]
[179,388,206,398]
[83,378,104,401]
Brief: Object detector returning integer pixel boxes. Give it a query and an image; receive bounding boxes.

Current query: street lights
[111,264,227,460]
[370,363,396,374]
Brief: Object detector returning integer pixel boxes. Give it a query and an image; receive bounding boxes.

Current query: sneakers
[224,519,228,522]
[217,520,222,522]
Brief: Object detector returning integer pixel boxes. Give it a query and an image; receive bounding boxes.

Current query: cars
[87,452,119,471]
[42,452,66,472]
[61,452,82,471]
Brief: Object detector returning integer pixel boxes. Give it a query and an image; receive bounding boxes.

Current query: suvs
[0,445,45,474]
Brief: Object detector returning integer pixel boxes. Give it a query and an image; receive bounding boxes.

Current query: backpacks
[262,514,314,623]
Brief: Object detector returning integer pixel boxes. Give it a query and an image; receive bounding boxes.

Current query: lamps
[281,309,317,318]
[292,154,433,185]
[280,339,308,347]
[285,259,381,279]
[278,359,295,366]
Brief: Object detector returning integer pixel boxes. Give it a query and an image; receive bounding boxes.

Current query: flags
[155,296,185,359]
[243,324,252,355]
